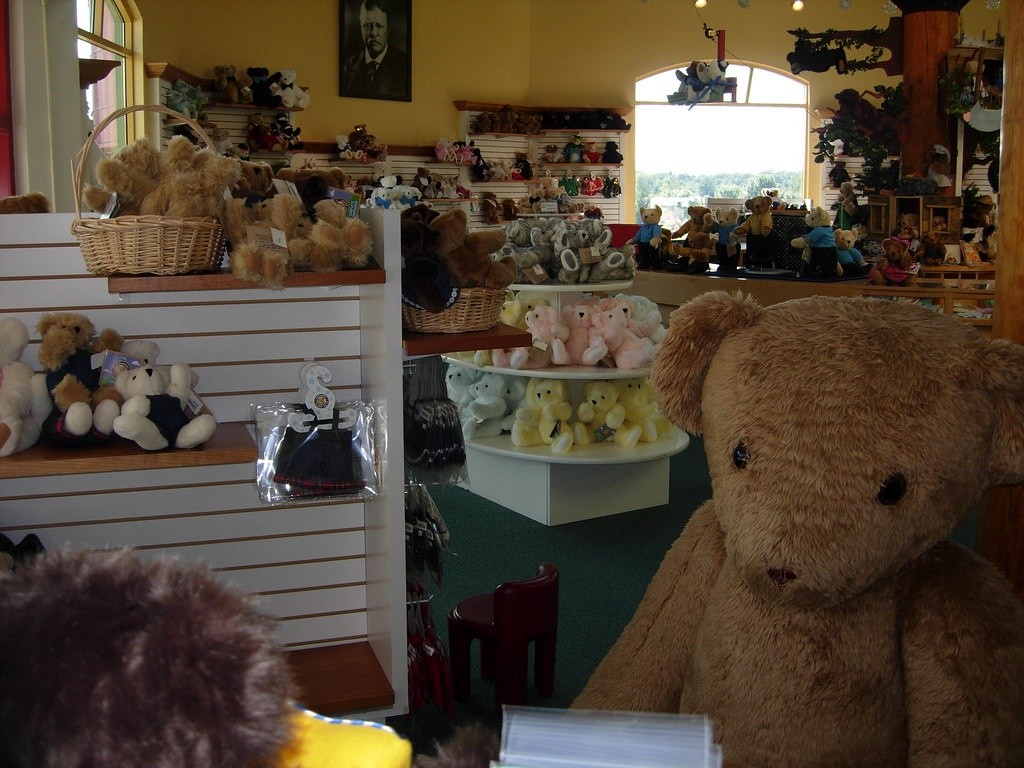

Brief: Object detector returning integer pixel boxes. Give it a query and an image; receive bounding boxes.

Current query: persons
[343,0,408,101]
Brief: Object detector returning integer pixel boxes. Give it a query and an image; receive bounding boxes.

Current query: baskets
[403,288,509,332]
[70,104,228,277]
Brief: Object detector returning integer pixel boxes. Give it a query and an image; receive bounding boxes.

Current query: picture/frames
[337,0,413,103]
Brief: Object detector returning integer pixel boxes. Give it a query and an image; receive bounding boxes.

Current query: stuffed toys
[225,158,374,291]
[399,204,519,313]
[412,166,473,200]
[84,134,241,218]
[625,182,996,286]
[0,544,304,768]
[666,59,729,105]
[470,103,632,132]
[541,134,624,165]
[828,161,851,187]
[445,363,677,458]
[787,32,850,75]
[416,723,500,768]
[568,291,1024,768]
[489,217,637,285]
[483,185,604,226]
[166,64,310,161]
[965,61,989,110]
[927,144,952,199]
[0,312,218,459]
[360,162,434,213]
[434,135,475,167]
[457,292,672,371]
[471,148,536,182]
[330,123,389,164]
[834,89,882,155]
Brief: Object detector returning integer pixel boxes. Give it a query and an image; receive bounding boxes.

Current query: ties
[369,61,378,81]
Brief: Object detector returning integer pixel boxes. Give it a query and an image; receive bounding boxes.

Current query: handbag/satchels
[254,398,388,510]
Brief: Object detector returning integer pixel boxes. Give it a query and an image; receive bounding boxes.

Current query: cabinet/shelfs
[444,213,690,528]
[290,140,459,214]
[0,204,531,726]
[453,99,634,233]
[144,61,310,168]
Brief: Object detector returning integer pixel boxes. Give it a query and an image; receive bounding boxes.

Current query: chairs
[447,564,562,717]
